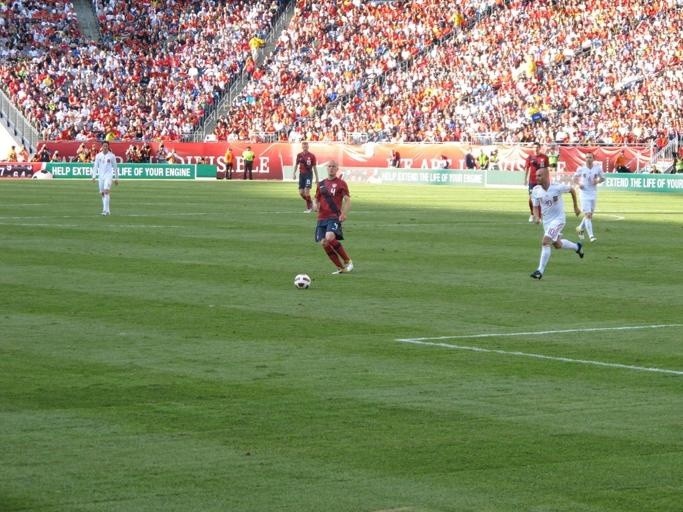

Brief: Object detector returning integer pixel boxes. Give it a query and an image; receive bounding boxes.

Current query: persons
[92,141,118,215]
[290,142,319,213]
[530,167,584,280]
[310,161,353,274]
[572,152,605,243]
[523,141,548,222]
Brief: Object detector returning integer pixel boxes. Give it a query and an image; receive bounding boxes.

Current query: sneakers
[575,226,597,242]
[528,214,542,225]
[331,259,354,275]
[303,200,316,214]
[576,242,584,258]
[101,210,110,217]
[530,270,542,280]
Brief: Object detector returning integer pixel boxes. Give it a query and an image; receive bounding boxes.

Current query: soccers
[295,274,311,289]
